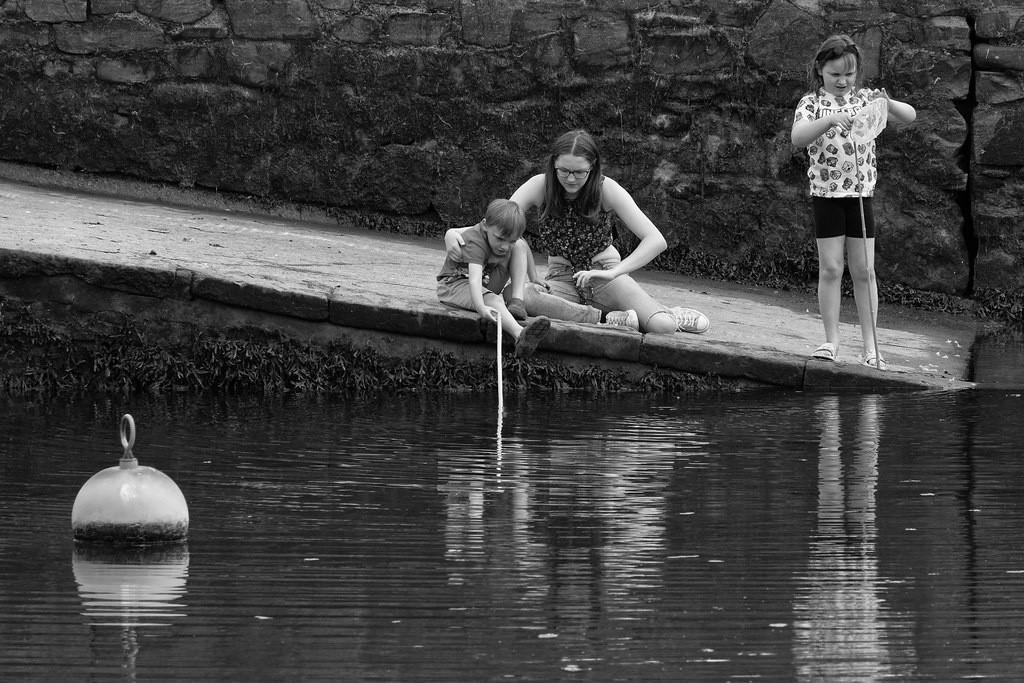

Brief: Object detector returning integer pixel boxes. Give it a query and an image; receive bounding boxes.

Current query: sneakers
[605,309,640,330]
[665,305,710,333]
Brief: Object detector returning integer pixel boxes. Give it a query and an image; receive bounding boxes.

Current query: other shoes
[513,314,551,356]
[506,298,527,321]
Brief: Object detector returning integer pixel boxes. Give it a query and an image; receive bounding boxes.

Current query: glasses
[553,163,593,180]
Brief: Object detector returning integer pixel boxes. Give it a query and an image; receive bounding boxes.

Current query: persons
[791,34,916,372]
[437,199,550,359]
[794,394,883,680]
[443,131,709,333]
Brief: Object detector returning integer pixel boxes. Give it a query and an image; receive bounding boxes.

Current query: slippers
[857,351,887,370]
[812,343,837,360]
